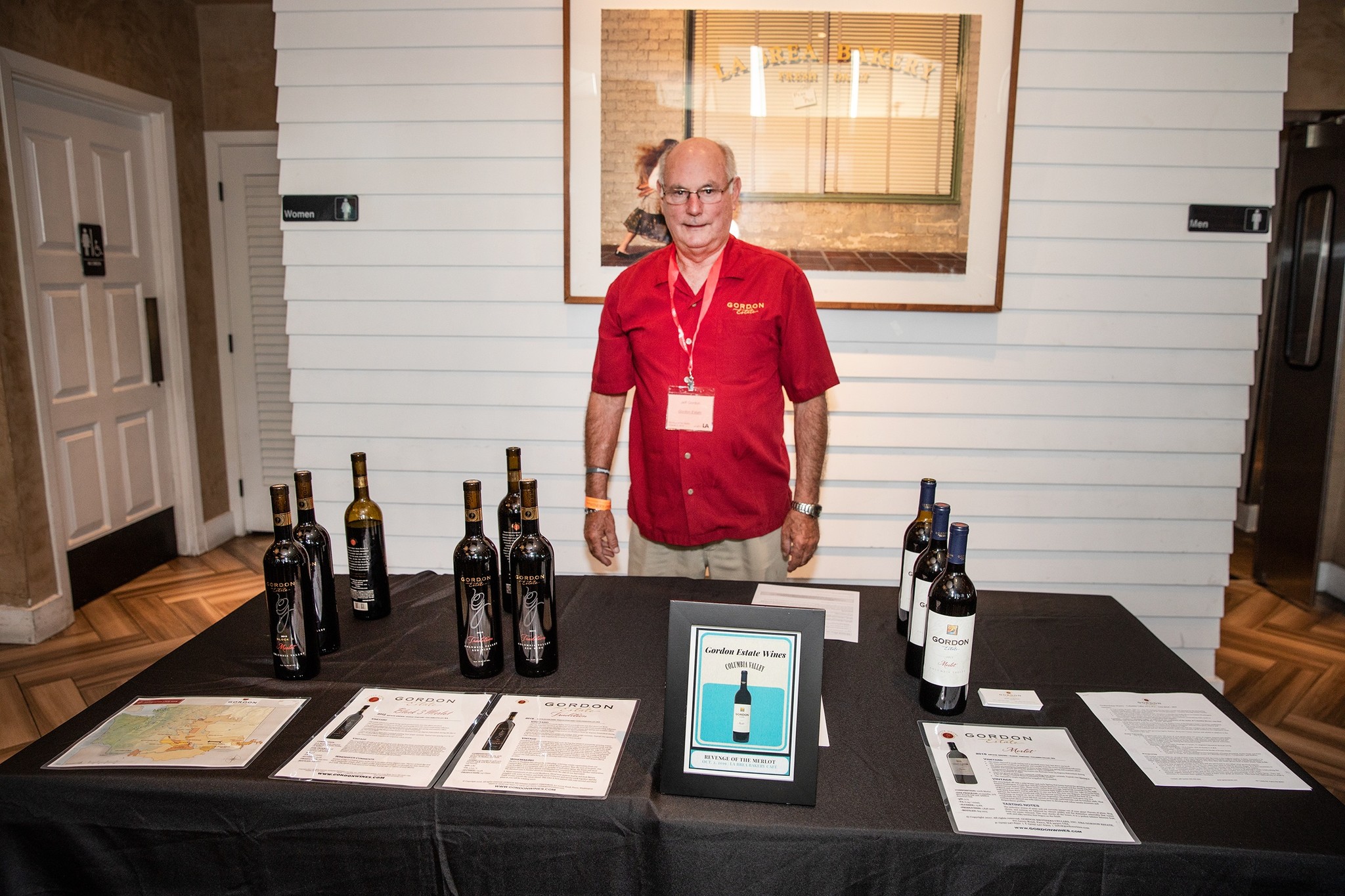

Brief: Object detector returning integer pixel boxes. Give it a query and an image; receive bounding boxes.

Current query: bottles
[732,670,752,742]
[265,446,561,678]
[482,711,519,751]
[897,479,975,715]
[326,705,370,739]
[946,742,978,784]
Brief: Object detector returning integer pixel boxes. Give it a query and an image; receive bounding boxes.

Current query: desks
[0,570,1345,896]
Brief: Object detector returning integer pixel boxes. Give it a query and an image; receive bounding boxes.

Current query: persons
[582,139,840,582]
[614,140,678,259]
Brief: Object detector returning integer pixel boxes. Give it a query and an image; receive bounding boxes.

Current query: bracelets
[584,508,599,514]
[585,467,612,476]
[583,496,611,511]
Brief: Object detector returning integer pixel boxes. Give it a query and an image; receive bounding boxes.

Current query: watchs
[791,500,823,518]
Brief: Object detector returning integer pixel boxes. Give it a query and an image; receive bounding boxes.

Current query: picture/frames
[556,1,1025,312]
[660,599,826,807]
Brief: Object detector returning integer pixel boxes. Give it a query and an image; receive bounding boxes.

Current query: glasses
[660,177,734,205]
